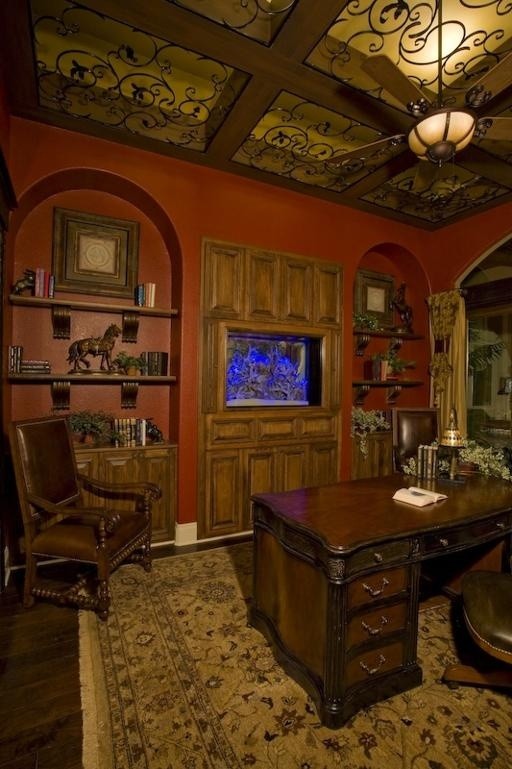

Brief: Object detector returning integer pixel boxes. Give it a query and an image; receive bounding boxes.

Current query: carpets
[74,541,510,768]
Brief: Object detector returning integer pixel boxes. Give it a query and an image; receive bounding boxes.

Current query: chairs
[390,407,441,473]
[3,415,162,622]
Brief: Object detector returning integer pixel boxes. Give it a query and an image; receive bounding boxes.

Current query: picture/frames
[48,206,140,299]
[351,268,396,328]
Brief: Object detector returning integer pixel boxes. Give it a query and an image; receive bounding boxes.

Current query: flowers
[350,407,390,457]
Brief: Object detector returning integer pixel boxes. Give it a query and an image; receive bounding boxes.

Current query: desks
[246,474,512,730]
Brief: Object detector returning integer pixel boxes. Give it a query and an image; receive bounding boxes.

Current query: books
[415,442,439,483]
[136,281,155,308]
[363,359,390,381]
[33,268,56,300]
[392,486,448,510]
[111,417,148,447]
[140,350,168,377]
[8,344,52,375]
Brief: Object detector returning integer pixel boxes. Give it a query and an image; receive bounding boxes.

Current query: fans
[321,0,512,174]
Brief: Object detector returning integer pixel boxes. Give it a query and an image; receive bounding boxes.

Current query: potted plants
[370,351,415,381]
[66,409,129,446]
[113,349,146,377]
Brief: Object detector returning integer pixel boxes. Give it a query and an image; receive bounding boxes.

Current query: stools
[443,558,512,692]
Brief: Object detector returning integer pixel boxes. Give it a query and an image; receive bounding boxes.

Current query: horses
[67,323,122,371]
[388,281,414,327]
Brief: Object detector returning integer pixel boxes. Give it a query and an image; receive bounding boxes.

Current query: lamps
[407,109,479,169]
[440,405,466,484]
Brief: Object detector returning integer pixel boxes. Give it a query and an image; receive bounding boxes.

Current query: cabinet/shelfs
[14,449,175,552]
[9,290,180,389]
[204,407,340,536]
[200,237,344,331]
[351,325,426,406]
